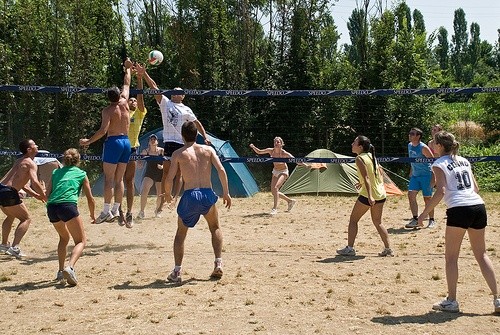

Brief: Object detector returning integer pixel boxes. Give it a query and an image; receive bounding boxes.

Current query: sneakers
[378,248,394,257]
[211,266,223,277]
[493,299,500,312]
[5,246,22,259]
[167,269,181,282]
[63,266,78,285]
[427,219,434,228]
[405,218,419,227]
[433,296,459,312]
[336,246,355,256]
[126,212,133,227]
[0,241,10,253]
[95,209,120,224]
[118,210,126,226]
[57,270,65,281]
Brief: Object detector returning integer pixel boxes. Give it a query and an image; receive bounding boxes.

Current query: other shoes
[287,199,297,213]
[270,207,277,215]
[137,210,145,219]
[153,209,161,218]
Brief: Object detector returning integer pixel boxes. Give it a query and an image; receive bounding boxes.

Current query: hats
[148,133,157,140]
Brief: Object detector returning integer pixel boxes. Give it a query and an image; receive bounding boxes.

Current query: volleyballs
[148,49,164,66]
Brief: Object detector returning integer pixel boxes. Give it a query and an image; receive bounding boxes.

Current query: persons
[428,124,441,156]
[0,138,45,257]
[80,60,133,224]
[133,62,211,213]
[44,148,97,285]
[137,134,167,219]
[165,122,232,280]
[250,137,309,215]
[405,128,435,228]
[117,62,148,229]
[418,132,500,314]
[336,136,396,255]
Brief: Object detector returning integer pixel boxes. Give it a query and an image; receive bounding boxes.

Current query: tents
[89,126,260,198]
[280,149,410,195]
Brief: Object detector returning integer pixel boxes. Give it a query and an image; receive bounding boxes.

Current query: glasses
[432,129,436,131]
[408,132,414,135]
